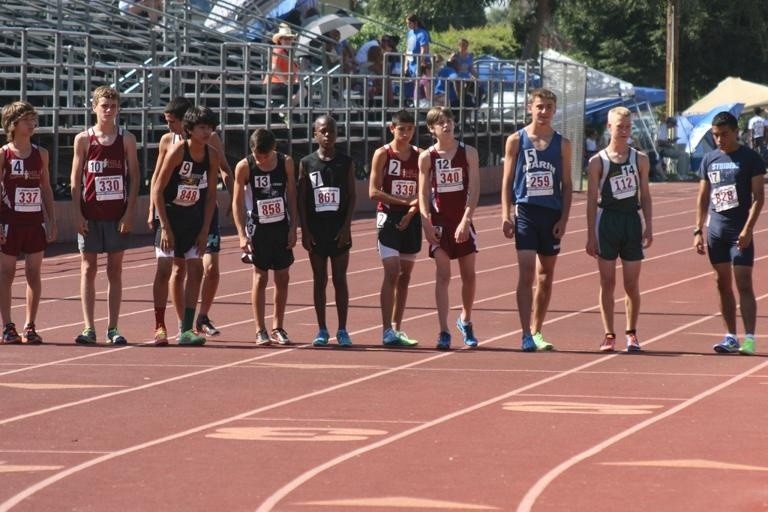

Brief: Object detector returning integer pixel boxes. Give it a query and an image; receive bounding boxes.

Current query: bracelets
[694,228,703,238]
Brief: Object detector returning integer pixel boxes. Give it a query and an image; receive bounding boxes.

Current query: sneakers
[20,325,42,343]
[0,322,23,347]
[74,328,96,346]
[256,331,270,346]
[712,332,756,355]
[625,330,640,352]
[337,330,351,346]
[105,328,124,346]
[524,332,555,353]
[177,321,220,345]
[457,317,478,346]
[154,326,168,348]
[598,329,616,353]
[437,330,450,350]
[270,328,292,345]
[312,330,329,346]
[382,328,418,348]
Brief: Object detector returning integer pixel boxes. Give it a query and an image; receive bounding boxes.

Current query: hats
[271,25,297,43]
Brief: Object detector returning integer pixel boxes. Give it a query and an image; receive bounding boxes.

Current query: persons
[582,127,601,177]
[151,103,220,347]
[229,126,300,349]
[692,111,765,355]
[496,88,573,354]
[148,93,235,338]
[417,107,485,352]
[582,103,657,352]
[654,116,695,182]
[364,108,426,349]
[116,2,163,31]
[263,8,488,140]
[0,99,56,346]
[296,113,360,349]
[67,86,142,346]
[745,105,768,164]
